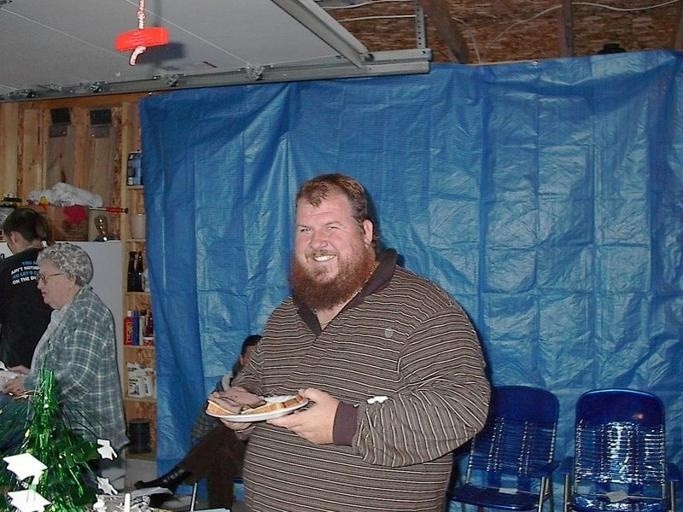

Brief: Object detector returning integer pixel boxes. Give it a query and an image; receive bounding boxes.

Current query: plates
[205,395,308,423]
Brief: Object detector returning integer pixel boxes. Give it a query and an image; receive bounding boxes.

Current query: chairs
[446,385,560,512]
[189,479,244,512]
[563,388,679,512]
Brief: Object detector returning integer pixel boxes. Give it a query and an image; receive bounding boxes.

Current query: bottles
[124,309,154,346]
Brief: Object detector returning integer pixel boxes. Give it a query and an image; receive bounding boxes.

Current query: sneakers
[133,476,177,507]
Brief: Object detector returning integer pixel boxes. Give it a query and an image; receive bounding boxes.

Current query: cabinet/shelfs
[122,186,156,452]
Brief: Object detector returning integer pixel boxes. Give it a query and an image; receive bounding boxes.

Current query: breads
[208,394,302,415]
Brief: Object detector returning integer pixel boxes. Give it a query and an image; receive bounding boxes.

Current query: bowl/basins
[0,372,27,392]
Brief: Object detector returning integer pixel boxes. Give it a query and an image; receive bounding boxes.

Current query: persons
[0,207,55,449]
[219,172,492,512]
[5,242,131,493]
[134,335,264,512]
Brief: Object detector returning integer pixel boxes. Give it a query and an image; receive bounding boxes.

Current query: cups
[126,250,143,290]
[131,210,147,241]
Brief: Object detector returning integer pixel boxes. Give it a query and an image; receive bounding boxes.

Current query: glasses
[34,272,64,285]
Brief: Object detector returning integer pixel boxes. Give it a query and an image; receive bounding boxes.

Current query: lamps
[116,0,168,66]
